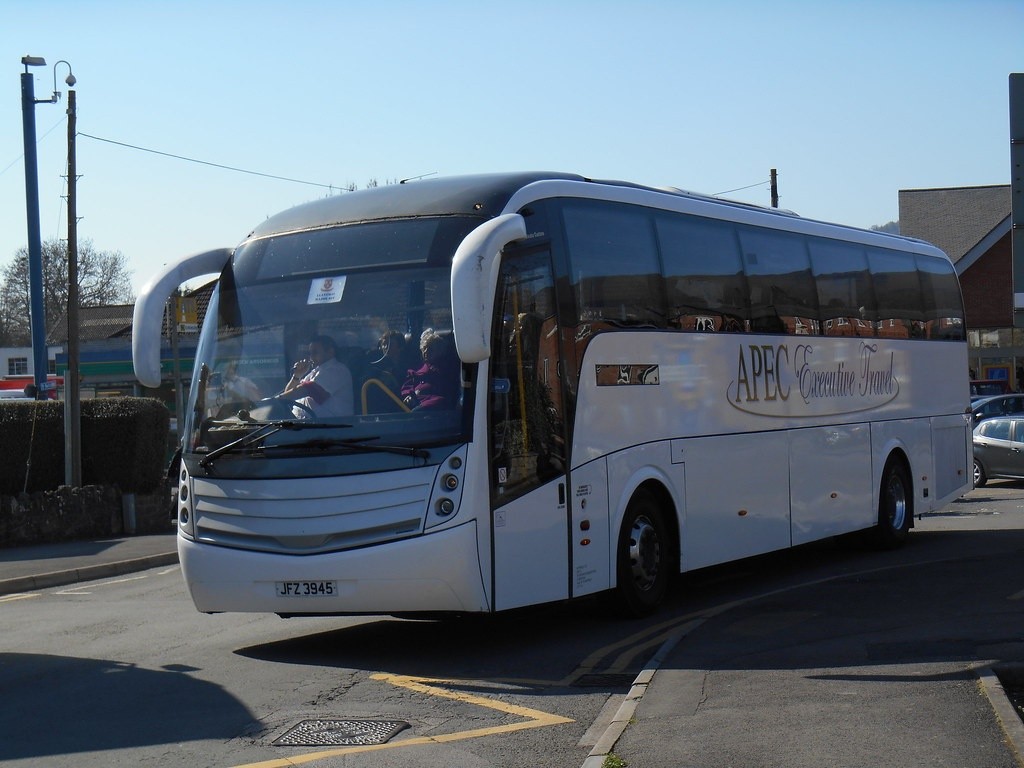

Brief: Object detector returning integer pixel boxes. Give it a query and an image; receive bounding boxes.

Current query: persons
[211,359,261,418]
[271,328,460,435]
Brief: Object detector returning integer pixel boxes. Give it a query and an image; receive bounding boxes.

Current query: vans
[970,379,1013,396]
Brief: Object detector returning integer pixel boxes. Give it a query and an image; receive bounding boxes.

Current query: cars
[971,394,1024,427]
[973,416,1024,487]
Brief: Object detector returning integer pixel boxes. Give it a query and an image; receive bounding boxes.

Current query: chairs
[418,330,455,367]
[379,334,411,365]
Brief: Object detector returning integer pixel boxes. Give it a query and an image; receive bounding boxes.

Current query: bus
[133,171,974,620]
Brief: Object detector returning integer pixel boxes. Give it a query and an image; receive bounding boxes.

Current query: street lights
[21,55,76,402]
